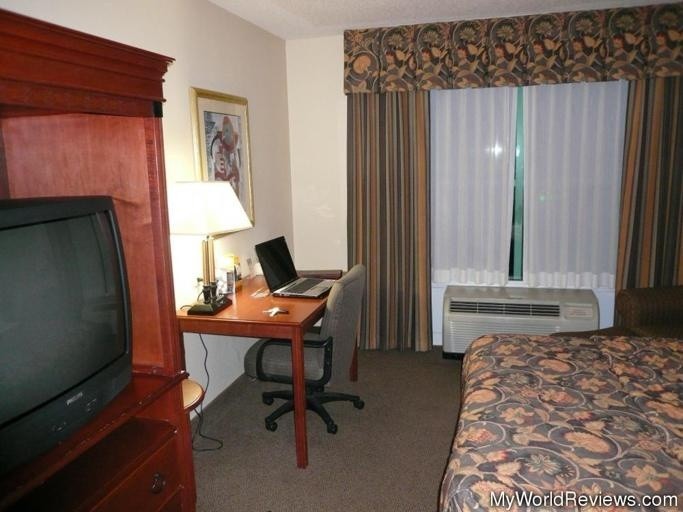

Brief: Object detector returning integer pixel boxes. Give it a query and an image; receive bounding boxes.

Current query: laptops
[255,235,340,299]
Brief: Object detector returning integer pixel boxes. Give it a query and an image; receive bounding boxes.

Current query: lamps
[167,180,253,319]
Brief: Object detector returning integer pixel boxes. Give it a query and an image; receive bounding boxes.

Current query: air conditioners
[439,283,602,361]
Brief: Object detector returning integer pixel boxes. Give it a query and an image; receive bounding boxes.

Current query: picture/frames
[187,83,258,241]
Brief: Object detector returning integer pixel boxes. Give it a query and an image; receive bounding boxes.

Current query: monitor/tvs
[0,194,134,478]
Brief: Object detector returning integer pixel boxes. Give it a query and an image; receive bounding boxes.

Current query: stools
[613,277,682,341]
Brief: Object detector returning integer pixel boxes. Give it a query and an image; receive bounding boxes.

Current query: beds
[442,331,683,512]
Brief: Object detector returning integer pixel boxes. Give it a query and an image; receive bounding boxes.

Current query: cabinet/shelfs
[1,9,195,512]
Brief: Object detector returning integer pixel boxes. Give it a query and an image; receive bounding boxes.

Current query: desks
[177,268,363,469]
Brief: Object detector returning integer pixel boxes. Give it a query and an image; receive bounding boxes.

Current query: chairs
[246,263,367,435]
